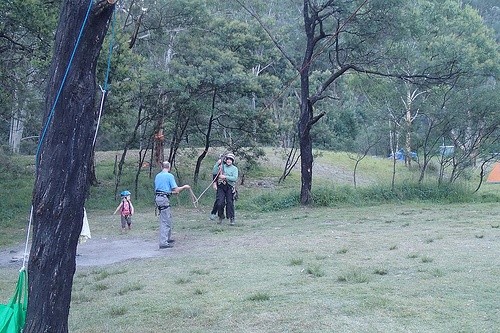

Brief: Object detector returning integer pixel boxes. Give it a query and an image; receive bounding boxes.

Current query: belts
[156,195,163,196]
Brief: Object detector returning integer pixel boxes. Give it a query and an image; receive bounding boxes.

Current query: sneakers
[229,217,235,226]
[210,209,217,220]
[216,217,222,223]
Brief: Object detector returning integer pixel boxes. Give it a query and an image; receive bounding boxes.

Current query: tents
[486,161,500,182]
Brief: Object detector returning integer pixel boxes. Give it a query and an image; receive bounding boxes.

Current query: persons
[154,161,190,248]
[114,190,134,233]
[209,154,238,225]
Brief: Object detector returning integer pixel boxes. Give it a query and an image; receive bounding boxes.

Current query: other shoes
[160,245,173,249]
[128,224,132,229]
[122,229,125,233]
[167,239,175,243]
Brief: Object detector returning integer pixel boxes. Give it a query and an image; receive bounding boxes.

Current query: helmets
[121,190,131,196]
[227,153,235,162]
